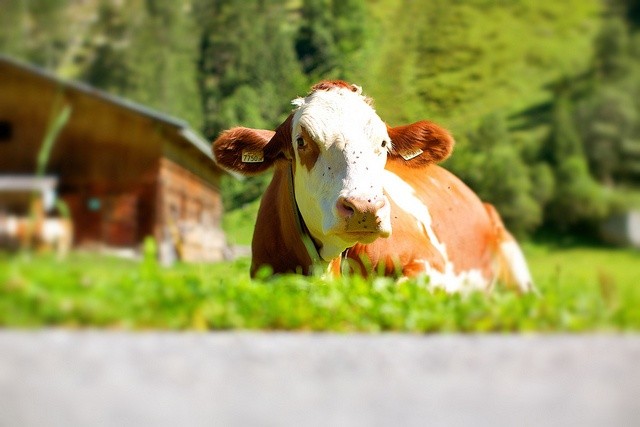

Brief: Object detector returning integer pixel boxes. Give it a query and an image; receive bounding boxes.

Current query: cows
[210,78,541,298]
